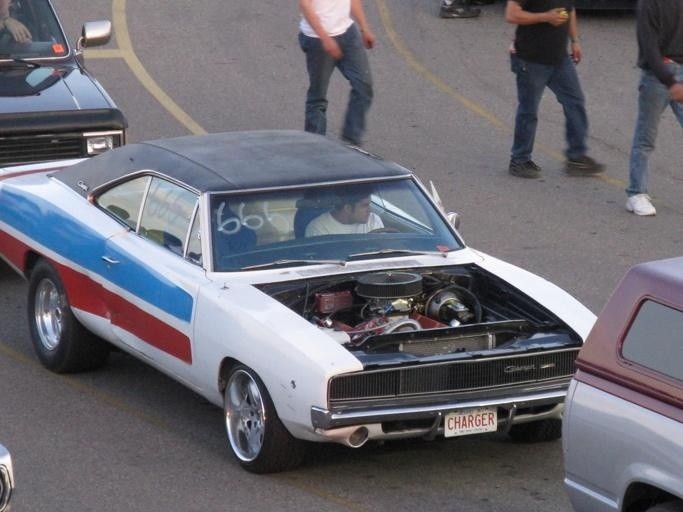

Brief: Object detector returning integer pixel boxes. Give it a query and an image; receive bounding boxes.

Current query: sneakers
[440,1,480,18]
[509,161,541,179]
[565,157,606,173]
[624,193,656,215]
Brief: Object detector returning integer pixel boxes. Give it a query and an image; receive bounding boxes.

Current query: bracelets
[568,36,583,43]
[2,16,11,30]
[360,27,368,33]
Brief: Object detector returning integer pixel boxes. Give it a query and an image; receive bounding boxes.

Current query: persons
[437,0,483,20]
[500,1,607,178]
[294,1,377,148]
[620,1,681,216]
[303,184,384,237]
[0,1,32,44]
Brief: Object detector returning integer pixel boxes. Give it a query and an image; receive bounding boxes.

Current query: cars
[0,443,14,512]
[0,0,129,171]
[0,129,596,474]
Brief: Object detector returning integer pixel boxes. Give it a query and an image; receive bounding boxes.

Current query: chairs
[295,192,335,239]
[162,194,256,262]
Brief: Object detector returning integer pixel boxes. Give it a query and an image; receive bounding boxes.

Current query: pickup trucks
[558,255,683,510]
